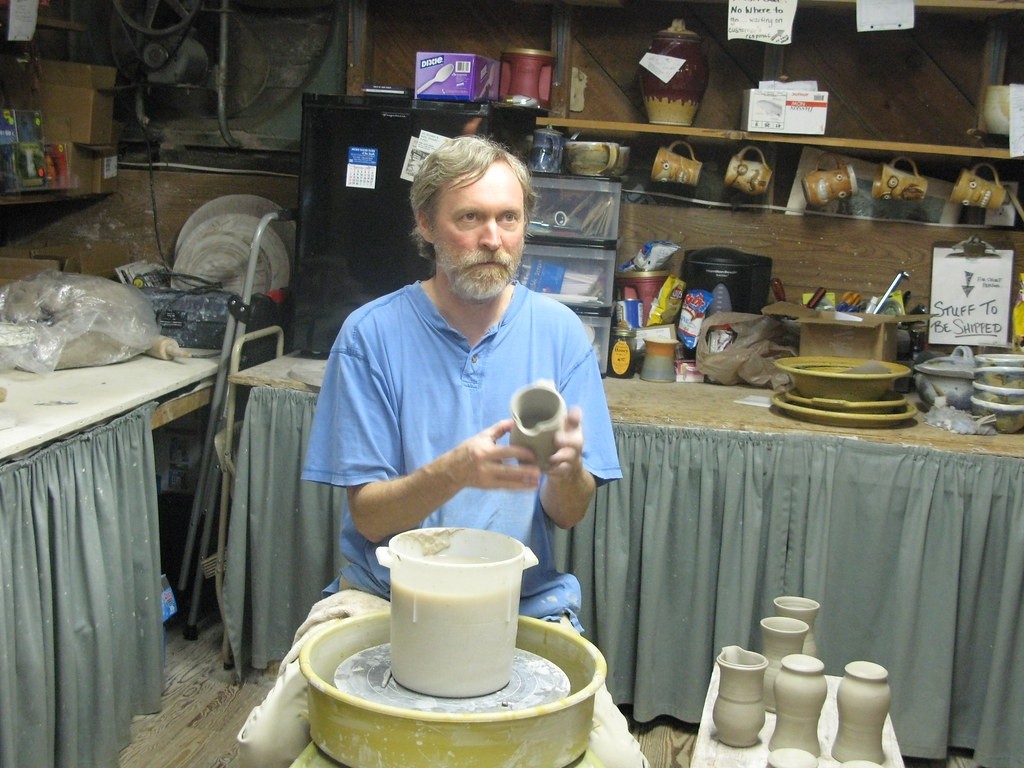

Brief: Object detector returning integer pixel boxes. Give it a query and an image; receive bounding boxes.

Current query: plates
[773,386,919,427]
[169,192,297,303]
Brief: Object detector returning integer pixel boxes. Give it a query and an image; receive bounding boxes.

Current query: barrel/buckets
[376,528,539,698]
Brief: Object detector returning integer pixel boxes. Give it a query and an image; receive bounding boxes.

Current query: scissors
[841,292,862,307]
[837,302,859,314]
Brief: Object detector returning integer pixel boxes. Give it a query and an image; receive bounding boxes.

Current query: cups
[531,125,773,196]
[801,151,859,207]
[950,163,1007,212]
[870,155,929,202]
[841,760,881,767]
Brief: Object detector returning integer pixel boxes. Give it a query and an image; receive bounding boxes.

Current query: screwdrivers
[771,279,785,302]
[806,287,826,309]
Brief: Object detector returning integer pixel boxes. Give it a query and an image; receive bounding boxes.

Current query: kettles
[684,246,784,320]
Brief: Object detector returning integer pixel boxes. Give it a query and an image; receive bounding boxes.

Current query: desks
[0,348,222,768]
[216,326,1024,768]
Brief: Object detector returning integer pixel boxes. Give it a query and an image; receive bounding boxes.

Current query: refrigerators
[298,91,549,358]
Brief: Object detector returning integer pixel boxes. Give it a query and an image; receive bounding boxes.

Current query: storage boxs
[801,308,938,364]
[414,51,499,103]
[570,309,610,382]
[0,53,128,194]
[742,89,829,134]
[524,174,624,246]
[514,240,616,313]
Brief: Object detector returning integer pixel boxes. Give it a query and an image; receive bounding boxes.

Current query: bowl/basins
[773,355,913,402]
[299,610,609,768]
[913,346,1023,433]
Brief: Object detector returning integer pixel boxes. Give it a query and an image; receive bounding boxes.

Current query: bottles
[616,267,667,326]
[638,17,711,127]
[832,660,890,765]
[497,47,555,109]
[766,748,819,768]
[774,594,820,657]
[760,617,810,713]
[609,325,681,383]
[768,654,828,758]
[864,296,879,314]
[506,381,568,472]
[712,651,769,747]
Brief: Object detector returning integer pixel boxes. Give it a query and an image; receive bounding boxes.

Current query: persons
[237,135,649,768]
